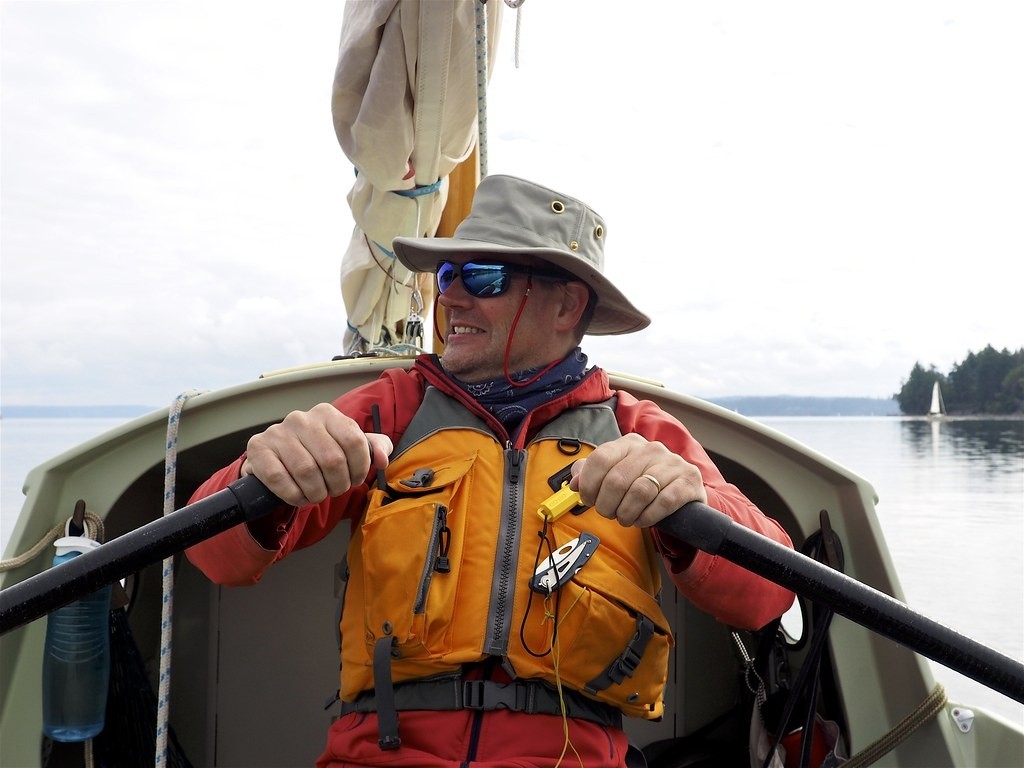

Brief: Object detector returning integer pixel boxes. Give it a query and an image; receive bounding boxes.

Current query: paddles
[652,497,1023,706]
[1,443,375,642]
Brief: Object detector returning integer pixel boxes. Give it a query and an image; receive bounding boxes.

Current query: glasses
[434,259,559,298]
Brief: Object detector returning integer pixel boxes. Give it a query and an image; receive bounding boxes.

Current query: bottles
[760,687,830,768]
[41,516,112,743]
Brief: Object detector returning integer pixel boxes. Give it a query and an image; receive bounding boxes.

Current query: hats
[392,173,651,337]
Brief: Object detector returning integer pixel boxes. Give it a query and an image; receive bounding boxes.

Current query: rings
[641,473,662,494]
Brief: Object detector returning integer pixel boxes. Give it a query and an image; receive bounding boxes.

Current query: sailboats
[926,379,948,417]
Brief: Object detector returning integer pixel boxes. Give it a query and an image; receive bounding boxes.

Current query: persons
[177,173,801,768]
